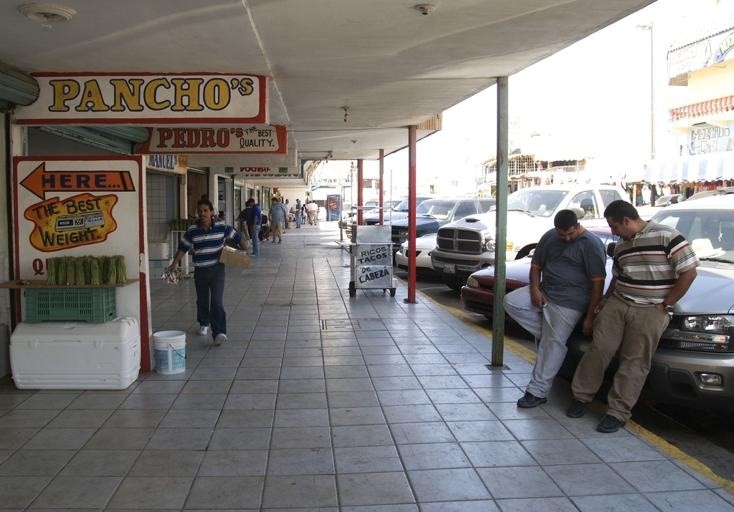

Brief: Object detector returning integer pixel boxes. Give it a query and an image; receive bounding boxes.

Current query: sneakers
[566,399,591,418]
[596,412,626,433]
[198,326,227,344]
[518,392,547,407]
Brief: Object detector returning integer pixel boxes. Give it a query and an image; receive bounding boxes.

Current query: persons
[164,197,251,349]
[564,199,699,435]
[502,209,606,410]
[238,196,319,258]
[579,198,594,218]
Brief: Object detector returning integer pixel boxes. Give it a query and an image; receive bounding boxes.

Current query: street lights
[635,19,657,162]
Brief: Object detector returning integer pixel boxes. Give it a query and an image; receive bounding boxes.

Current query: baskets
[24,288,116,324]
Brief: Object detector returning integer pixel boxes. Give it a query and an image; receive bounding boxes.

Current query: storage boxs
[219,246,250,269]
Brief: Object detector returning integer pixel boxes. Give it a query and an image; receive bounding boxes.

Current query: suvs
[607,192,734,414]
[430,183,632,292]
[346,198,401,240]
[374,196,496,254]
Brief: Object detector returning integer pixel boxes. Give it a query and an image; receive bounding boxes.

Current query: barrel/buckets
[153,330,186,376]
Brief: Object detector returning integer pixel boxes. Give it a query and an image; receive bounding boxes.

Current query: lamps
[339,104,350,123]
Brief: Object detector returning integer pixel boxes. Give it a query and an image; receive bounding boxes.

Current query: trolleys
[349,224,398,297]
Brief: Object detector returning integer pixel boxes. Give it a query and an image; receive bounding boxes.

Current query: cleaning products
[669,95,733,121]
[635,180,734,205]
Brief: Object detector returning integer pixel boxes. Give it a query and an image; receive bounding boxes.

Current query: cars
[395,231,442,284]
[635,184,733,218]
[460,224,619,341]
[338,200,380,230]
